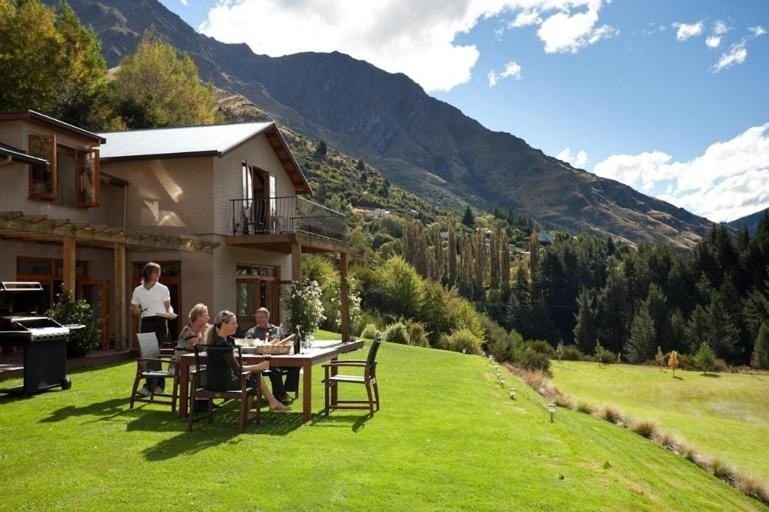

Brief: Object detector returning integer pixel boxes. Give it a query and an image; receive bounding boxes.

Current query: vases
[63,340,87,360]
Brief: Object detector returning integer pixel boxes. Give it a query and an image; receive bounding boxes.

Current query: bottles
[294,325,301,355]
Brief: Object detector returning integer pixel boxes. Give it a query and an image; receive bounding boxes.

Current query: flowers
[45,279,103,351]
[279,275,328,334]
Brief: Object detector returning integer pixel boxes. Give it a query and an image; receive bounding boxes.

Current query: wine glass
[262,345,272,373]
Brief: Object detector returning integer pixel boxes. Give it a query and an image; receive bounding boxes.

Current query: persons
[243,305,300,403]
[204,308,290,411]
[130,261,177,397]
[166,302,216,381]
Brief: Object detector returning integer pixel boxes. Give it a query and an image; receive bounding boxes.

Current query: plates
[155,312,178,320]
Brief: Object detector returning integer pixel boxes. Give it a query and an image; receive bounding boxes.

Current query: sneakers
[135,387,164,398]
[280,397,292,403]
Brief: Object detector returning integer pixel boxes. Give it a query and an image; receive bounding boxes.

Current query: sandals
[269,402,292,412]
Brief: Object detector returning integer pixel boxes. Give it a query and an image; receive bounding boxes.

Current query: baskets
[256,345,292,355]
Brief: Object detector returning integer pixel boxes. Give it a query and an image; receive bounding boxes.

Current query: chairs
[130,330,382,432]
